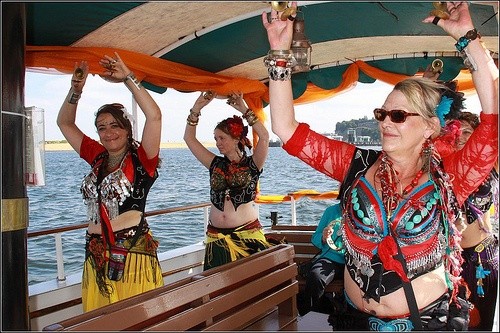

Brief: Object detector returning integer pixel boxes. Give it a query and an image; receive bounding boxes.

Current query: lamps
[290,7,312,74]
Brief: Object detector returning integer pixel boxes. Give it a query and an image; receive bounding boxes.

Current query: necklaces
[375,151,428,219]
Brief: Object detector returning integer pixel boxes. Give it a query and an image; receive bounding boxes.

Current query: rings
[207,98,210,100]
[432,16,440,25]
[270,18,279,21]
[288,15,295,21]
[449,7,456,12]
[109,72,113,76]
[104,59,116,70]
[457,2,463,7]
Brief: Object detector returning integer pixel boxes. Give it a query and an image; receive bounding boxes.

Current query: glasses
[374,108,421,123]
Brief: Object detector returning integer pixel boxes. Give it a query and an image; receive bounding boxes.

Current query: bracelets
[124,72,142,93]
[69,93,81,104]
[264,50,297,81]
[454,28,481,52]
[187,108,201,126]
[249,117,259,126]
[242,108,255,120]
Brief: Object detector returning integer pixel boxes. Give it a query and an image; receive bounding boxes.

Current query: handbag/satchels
[107,247,128,281]
[428,293,474,332]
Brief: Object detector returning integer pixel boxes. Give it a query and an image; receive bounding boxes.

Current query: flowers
[227,115,244,137]
[120,108,136,125]
[435,96,453,127]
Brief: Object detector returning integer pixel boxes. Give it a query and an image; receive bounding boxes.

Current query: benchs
[42,244,334,331]
[264,225,345,293]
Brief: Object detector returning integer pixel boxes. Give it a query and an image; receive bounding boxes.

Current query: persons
[302,204,350,315]
[56,51,165,315]
[454,112,499,332]
[262,1,499,333]
[183,91,271,272]
